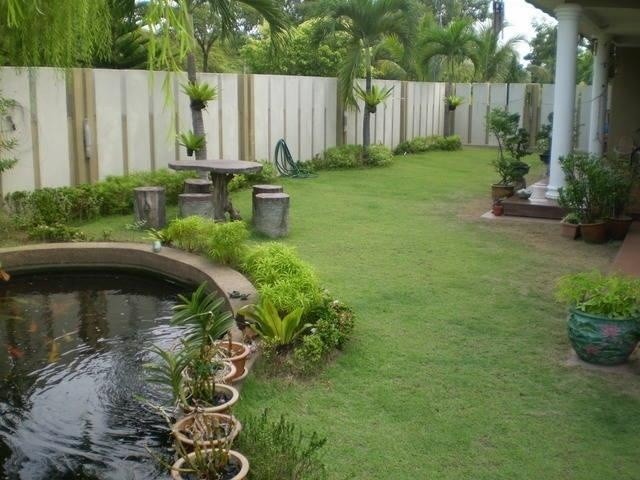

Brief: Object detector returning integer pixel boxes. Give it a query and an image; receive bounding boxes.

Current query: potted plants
[443,95,463,110]
[353,82,394,113]
[140,279,253,480]
[175,129,206,156]
[180,81,219,110]
[551,271,639,364]
[556,147,640,245]
[482,108,551,200]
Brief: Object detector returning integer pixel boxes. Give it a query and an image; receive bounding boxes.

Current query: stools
[133,178,291,240]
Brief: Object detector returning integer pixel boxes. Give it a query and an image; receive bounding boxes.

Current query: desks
[168,158,263,223]
